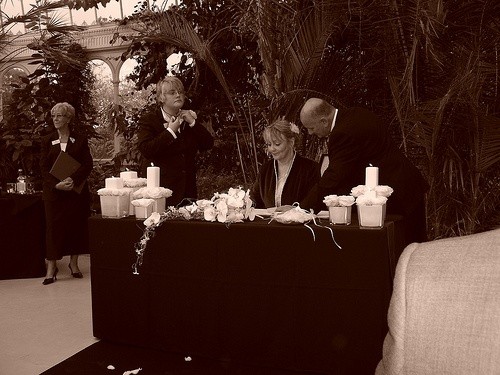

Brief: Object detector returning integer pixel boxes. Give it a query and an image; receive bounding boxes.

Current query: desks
[0,192,47,280]
[87,214,403,366]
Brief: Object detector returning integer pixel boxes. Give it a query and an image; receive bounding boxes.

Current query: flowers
[97,178,173,208]
[323,195,355,207]
[132,185,255,276]
[350,185,394,206]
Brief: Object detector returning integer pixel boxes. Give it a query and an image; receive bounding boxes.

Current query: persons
[136,75,214,210]
[250,98,500,375]
[37,103,94,284]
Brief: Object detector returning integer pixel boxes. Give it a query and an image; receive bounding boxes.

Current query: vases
[153,198,166,212]
[358,203,386,227]
[100,196,130,219]
[135,206,152,220]
[328,206,352,225]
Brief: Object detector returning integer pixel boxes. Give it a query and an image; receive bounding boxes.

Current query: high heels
[43,268,59,285]
[68,263,84,278]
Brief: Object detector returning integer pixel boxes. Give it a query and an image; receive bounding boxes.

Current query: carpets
[39,342,380,375]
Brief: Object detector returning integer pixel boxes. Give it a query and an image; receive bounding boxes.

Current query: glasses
[52,115,66,118]
[168,91,183,95]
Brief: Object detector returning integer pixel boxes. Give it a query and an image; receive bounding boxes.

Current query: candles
[147,162,160,186]
[120,168,137,178]
[366,163,378,185]
[105,176,123,190]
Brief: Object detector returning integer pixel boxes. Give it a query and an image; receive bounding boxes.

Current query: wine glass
[27,183,34,193]
